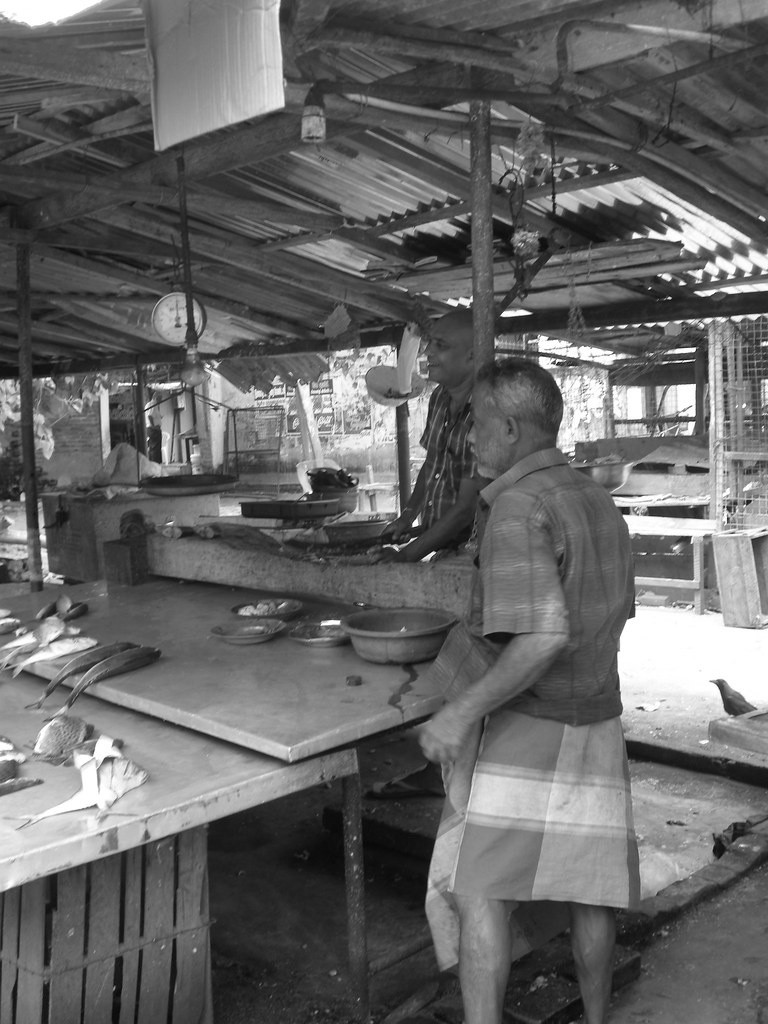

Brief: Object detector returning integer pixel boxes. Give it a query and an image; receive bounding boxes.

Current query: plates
[288,624,348,648]
[231,597,303,620]
[210,619,287,644]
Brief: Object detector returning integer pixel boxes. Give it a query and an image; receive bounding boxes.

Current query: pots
[323,520,389,544]
[310,485,360,514]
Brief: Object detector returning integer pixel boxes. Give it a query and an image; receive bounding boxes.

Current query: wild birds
[709,678,758,718]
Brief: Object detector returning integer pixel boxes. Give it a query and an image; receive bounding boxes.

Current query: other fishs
[0,614,98,678]
[24,638,143,711]
[42,646,162,722]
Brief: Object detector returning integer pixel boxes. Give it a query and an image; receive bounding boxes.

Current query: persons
[366,308,478,566]
[145,392,163,464]
[417,357,644,1024]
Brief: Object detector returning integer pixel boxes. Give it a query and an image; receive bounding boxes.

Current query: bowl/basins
[342,607,459,664]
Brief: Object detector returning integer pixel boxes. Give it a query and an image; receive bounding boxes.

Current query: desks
[622,514,721,615]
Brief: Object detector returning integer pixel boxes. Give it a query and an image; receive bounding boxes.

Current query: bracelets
[402,507,420,513]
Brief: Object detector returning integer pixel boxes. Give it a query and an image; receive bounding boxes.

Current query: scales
[130,230,246,497]
